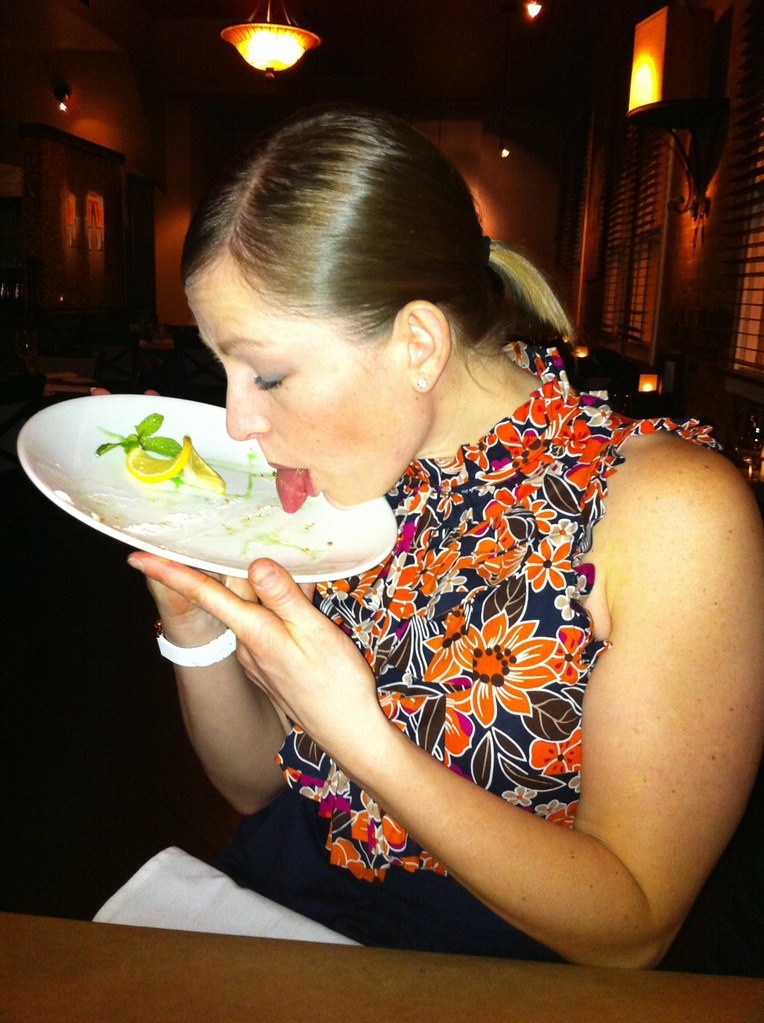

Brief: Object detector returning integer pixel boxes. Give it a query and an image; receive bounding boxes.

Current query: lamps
[219,0,321,79]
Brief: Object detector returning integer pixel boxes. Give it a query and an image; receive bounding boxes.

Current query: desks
[0,912,763,1023]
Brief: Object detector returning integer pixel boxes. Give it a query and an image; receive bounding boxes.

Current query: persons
[127,113,764,982]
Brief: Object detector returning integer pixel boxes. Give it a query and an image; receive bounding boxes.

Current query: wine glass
[733,409,764,483]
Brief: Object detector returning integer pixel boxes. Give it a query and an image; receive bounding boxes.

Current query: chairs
[0,350,105,472]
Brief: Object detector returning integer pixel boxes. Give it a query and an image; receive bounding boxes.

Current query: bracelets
[153,620,237,668]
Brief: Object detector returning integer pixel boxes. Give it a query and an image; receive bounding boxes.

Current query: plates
[17,394,398,583]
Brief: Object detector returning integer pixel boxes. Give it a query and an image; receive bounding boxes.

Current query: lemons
[127,436,226,495]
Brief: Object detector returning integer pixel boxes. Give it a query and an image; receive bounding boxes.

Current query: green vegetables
[96,412,183,457]
